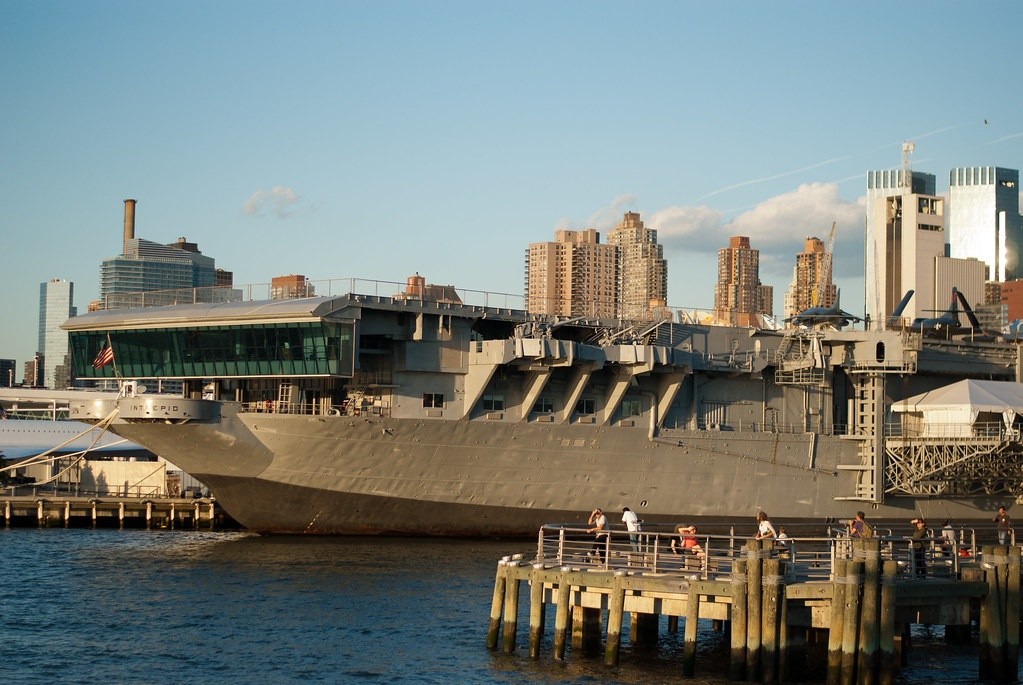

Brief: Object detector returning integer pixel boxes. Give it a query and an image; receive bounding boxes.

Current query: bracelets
[591,529,593,531]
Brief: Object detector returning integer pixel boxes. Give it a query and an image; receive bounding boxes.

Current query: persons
[587,508,609,564]
[668,524,705,569]
[265,398,270,413]
[937,521,956,556]
[847,511,867,538]
[992,506,1012,545]
[911,518,927,580]
[754,511,777,546]
[621,507,638,552]
[776,527,787,553]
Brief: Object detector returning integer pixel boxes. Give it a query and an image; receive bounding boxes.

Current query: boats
[67,193,1023,548]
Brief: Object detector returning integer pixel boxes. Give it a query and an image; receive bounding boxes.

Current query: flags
[93,337,115,369]
